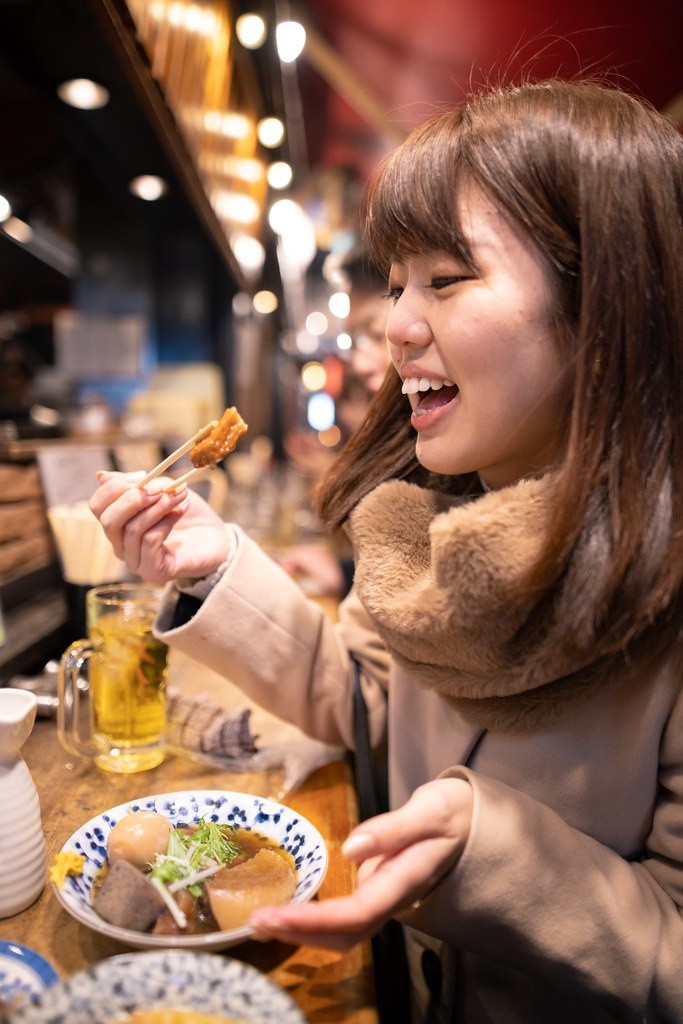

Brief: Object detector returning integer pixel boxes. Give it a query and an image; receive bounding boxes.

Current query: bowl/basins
[52,789,329,952]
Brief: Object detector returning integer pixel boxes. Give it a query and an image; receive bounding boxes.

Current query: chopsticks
[131,421,220,493]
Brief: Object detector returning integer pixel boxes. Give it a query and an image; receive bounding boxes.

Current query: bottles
[0,689,47,919]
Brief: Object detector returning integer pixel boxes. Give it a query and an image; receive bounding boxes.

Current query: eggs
[107,813,171,870]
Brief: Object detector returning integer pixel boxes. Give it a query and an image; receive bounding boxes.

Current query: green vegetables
[148,817,240,897]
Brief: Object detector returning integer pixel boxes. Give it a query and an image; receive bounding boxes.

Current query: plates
[0,949,306,1024]
[0,941,60,1022]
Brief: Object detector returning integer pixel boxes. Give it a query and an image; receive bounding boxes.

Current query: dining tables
[1,536,380,1023]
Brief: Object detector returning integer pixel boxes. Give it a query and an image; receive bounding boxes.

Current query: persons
[85,78,683,1023]
[263,242,390,624]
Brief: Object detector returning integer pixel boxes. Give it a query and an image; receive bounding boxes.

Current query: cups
[57,583,169,773]
